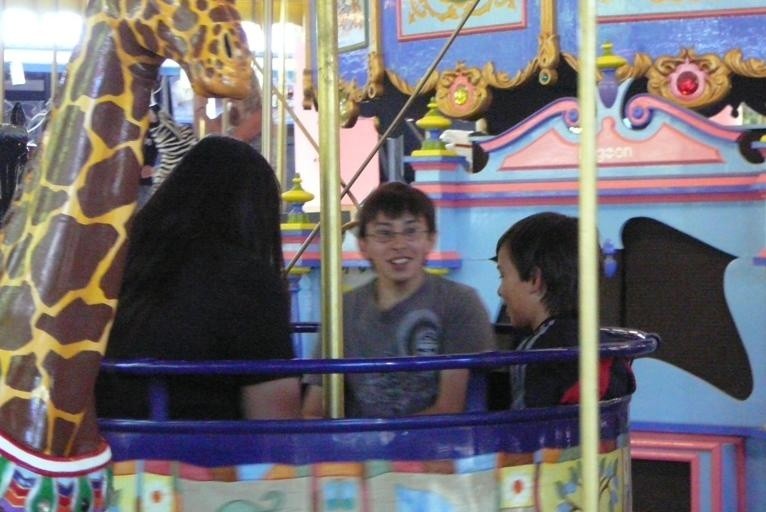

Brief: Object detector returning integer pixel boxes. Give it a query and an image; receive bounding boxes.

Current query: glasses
[365,227,431,244]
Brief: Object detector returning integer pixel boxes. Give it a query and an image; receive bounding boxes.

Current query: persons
[90,133,304,425]
[22,96,52,163]
[483,207,636,414]
[10,99,29,161]
[301,178,509,422]
[196,91,263,157]
[121,105,161,210]
